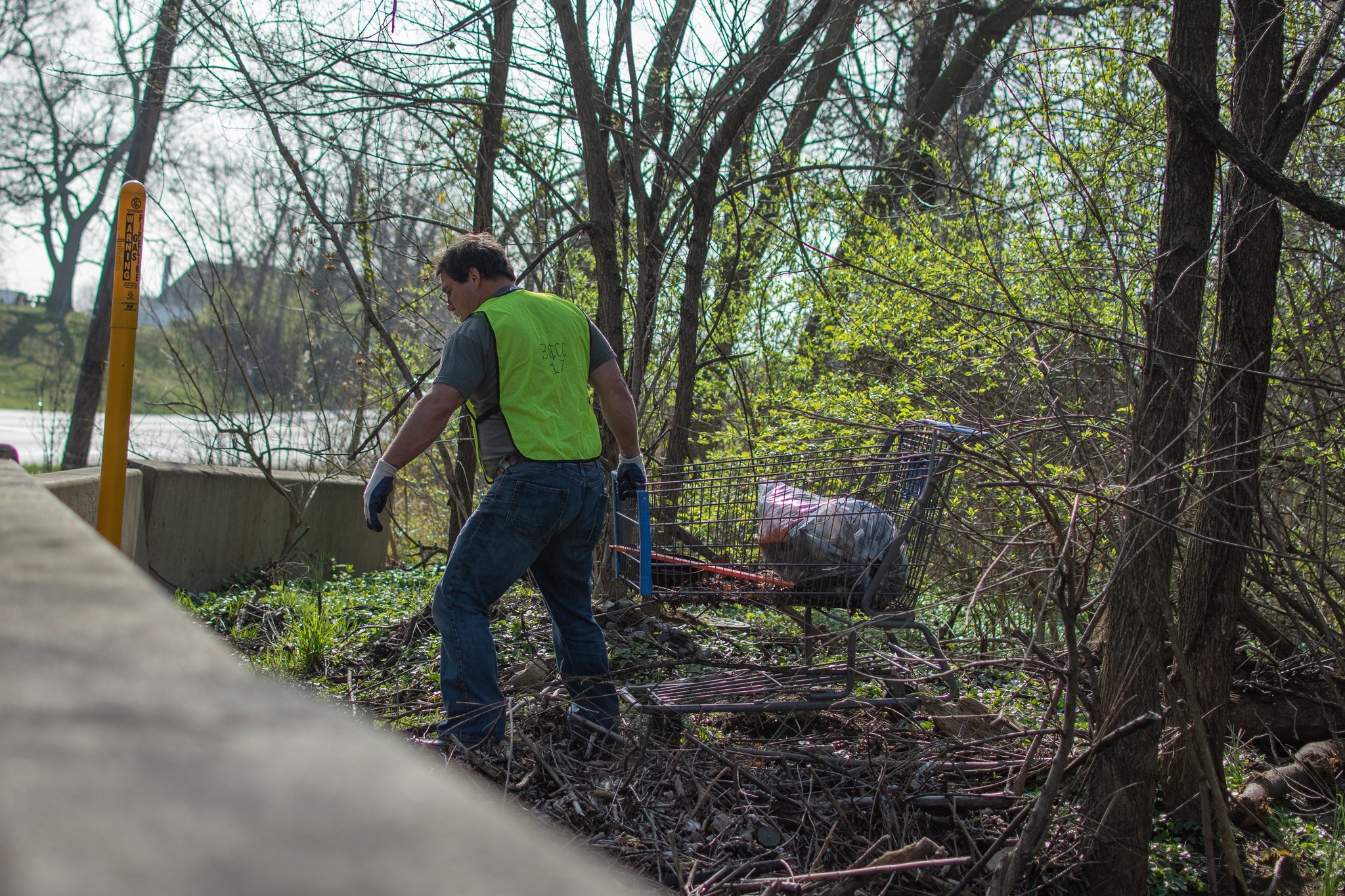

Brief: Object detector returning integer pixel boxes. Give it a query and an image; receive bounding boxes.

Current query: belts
[496,458,602,476]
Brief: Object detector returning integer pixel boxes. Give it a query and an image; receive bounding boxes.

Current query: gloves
[363,459,399,532]
[617,454,647,501]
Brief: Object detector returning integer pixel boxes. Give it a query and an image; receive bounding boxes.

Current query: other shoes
[408,736,483,752]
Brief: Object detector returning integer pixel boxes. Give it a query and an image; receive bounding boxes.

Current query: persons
[363,231,648,751]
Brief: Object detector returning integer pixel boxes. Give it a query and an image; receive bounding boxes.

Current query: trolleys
[608,418,992,736]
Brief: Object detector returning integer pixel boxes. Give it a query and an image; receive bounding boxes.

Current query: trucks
[0,290,54,312]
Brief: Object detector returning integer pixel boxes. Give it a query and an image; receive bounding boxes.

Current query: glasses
[439,271,465,305]
